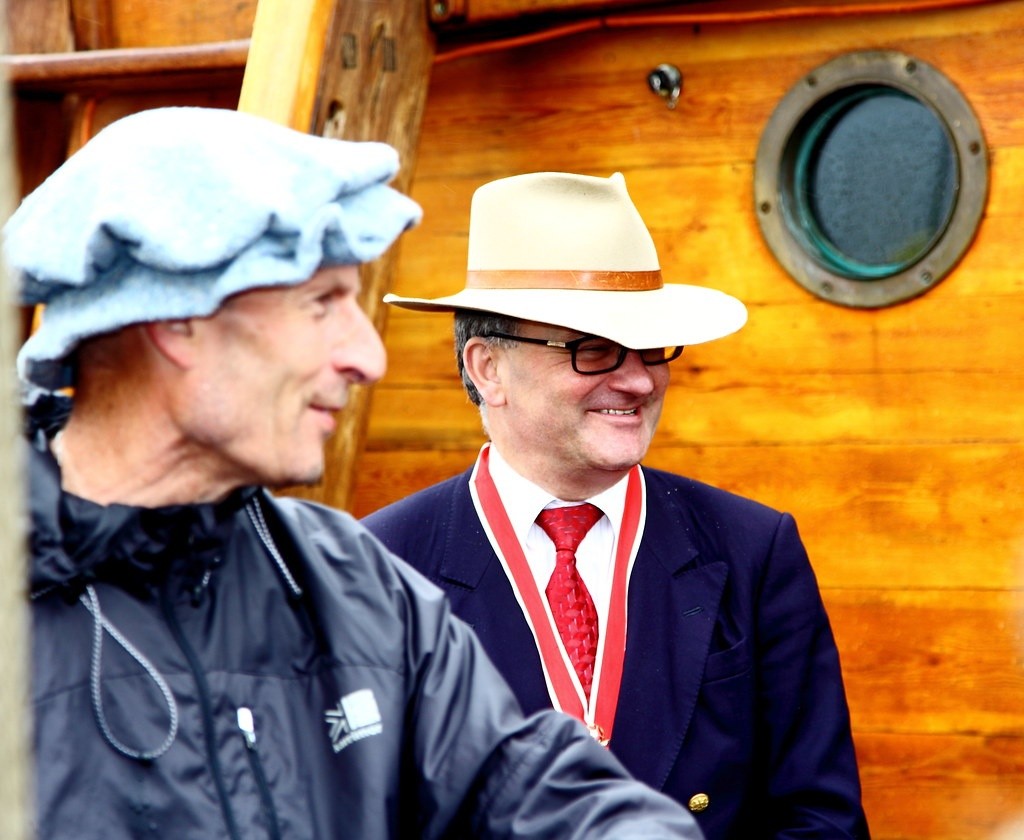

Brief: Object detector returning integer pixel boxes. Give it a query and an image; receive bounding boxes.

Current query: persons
[0,104,705,840]
[356,169,872,840]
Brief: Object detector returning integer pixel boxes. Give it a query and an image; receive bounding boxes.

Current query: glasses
[474,330,686,374]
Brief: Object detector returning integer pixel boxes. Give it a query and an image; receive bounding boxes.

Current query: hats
[8,105,421,391]
[379,170,749,350]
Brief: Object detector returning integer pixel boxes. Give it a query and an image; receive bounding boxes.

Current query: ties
[535,502,607,704]
